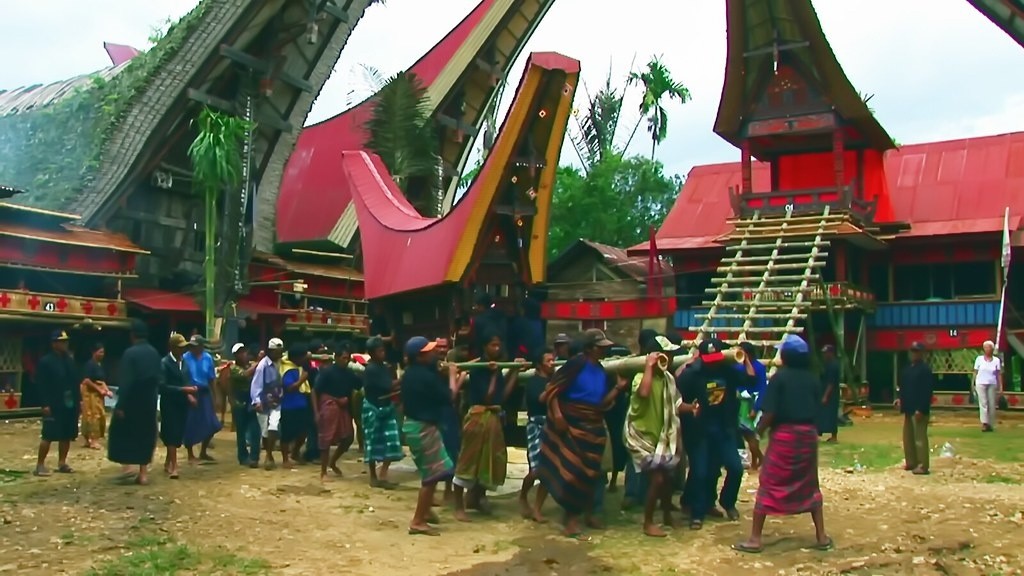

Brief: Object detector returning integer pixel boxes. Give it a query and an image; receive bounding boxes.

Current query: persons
[970,340,1004,432]
[544,328,630,538]
[34,331,80,476]
[78,319,842,552]
[892,341,934,475]
[401,337,463,536]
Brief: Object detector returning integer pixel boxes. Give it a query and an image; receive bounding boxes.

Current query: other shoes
[981,423,991,432]
[913,465,929,475]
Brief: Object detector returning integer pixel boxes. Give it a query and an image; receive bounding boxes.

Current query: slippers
[34,451,836,553]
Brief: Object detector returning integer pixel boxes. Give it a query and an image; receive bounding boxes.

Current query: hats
[773,335,808,355]
[268,338,283,349]
[51,329,69,340]
[646,335,681,351]
[553,333,570,344]
[232,343,244,354]
[698,337,725,362]
[820,345,834,352]
[169,334,191,346]
[910,341,925,352]
[405,336,437,357]
[188,334,205,346]
[584,329,614,346]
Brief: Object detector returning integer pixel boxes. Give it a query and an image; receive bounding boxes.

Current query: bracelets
[614,385,622,391]
